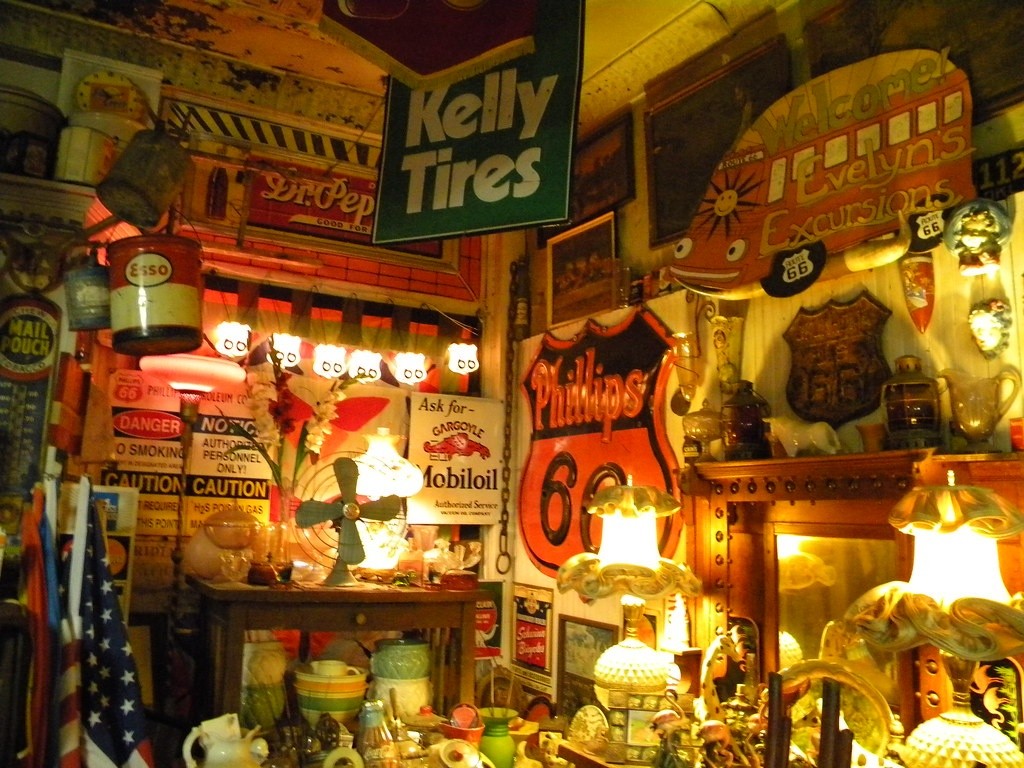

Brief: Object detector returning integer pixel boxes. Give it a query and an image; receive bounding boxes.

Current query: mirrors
[765,522,915,738]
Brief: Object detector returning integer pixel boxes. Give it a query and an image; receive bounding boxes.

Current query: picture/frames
[547,212,618,330]
[806,0,1024,126]
[537,113,637,248]
[644,34,793,250]
[606,691,702,768]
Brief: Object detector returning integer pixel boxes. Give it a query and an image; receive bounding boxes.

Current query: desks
[184,573,490,718]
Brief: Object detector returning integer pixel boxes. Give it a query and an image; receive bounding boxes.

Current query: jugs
[183,716,269,768]
[938,369,1020,452]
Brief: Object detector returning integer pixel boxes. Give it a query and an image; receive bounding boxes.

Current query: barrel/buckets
[106,209,205,356]
[53,124,116,188]
[96,127,194,227]
[63,267,111,332]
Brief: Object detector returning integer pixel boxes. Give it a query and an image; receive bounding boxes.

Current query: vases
[481,708,518,768]
[268,487,294,567]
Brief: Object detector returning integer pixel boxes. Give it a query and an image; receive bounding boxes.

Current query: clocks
[77,71,146,120]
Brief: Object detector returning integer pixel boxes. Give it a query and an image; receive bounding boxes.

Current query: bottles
[359,699,398,768]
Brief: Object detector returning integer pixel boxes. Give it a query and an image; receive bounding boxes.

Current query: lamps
[719,380,771,461]
[556,474,702,763]
[330,428,424,584]
[139,354,247,716]
[845,471,1024,768]
[880,355,943,448]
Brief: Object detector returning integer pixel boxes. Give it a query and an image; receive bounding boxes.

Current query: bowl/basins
[293,666,369,730]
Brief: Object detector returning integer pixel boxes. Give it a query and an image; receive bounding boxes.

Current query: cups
[311,659,361,677]
[370,638,431,679]
[218,550,252,581]
[375,678,433,717]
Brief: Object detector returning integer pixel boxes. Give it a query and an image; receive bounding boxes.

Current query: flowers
[216,334,373,561]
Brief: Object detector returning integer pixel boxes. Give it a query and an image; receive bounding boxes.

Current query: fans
[289,451,412,588]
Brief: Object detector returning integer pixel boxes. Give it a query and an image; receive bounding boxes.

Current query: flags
[14,473,154,768]
